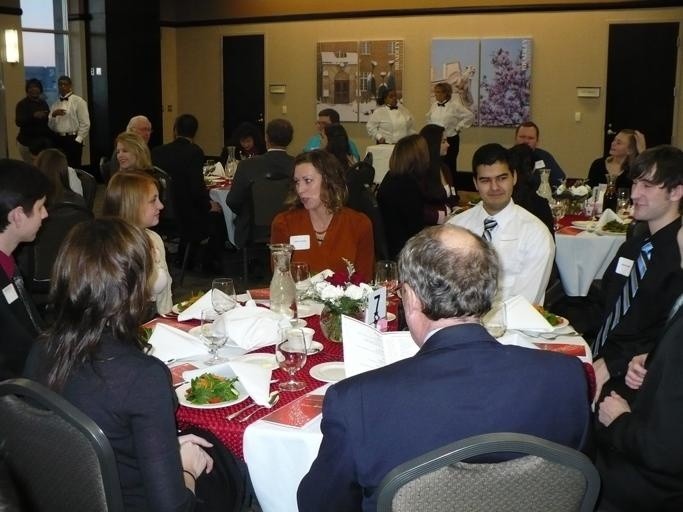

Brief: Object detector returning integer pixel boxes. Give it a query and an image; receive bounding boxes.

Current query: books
[340,313,420,379]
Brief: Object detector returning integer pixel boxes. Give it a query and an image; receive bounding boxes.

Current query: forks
[226,390,279,421]
[520,331,583,338]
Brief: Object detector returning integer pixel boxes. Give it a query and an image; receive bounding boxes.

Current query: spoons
[545,332,574,341]
[238,394,279,423]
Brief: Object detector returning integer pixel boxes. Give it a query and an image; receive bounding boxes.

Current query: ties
[14,277,39,334]
[481,218,497,242]
[589,241,654,358]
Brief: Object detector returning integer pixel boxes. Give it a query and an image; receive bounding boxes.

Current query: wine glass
[616,200,629,226]
[616,188,629,216]
[211,278,237,315]
[375,262,398,307]
[275,327,307,393]
[206,159,215,178]
[480,300,507,339]
[224,164,234,187]
[200,311,228,366]
[290,262,311,305]
[499,326,501,327]
[551,204,564,232]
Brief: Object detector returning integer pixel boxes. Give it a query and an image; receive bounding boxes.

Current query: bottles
[538,169,554,204]
[224,147,238,179]
[269,243,296,322]
[602,174,618,214]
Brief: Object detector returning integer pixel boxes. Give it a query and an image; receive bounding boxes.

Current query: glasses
[394,283,406,296]
[59,83,70,85]
[317,121,327,126]
[134,127,151,132]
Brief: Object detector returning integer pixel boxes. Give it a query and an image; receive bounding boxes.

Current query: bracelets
[183,465,197,480]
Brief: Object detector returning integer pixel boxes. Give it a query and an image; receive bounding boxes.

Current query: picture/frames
[315,40,404,124]
[431,37,532,127]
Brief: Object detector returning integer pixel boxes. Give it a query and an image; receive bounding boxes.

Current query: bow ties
[437,98,449,107]
[386,103,398,110]
[60,93,73,101]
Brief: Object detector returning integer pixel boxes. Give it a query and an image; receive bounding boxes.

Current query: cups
[285,328,316,350]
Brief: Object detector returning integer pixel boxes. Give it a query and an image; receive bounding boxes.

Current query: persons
[17,148,88,290]
[575,145,683,411]
[150,113,229,238]
[418,123,459,214]
[237,129,267,160]
[270,148,374,282]
[1,159,49,378]
[425,81,474,199]
[446,142,557,308]
[20,217,244,511]
[111,115,152,175]
[102,173,172,322]
[15,78,50,162]
[47,75,90,169]
[319,124,356,168]
[302,109,361,164]
[509,144,564,291]
[587,129,646,188]
[226,117,298,276]
[378,135,449,254]
[365,89,414,145]
[515,121,567,181]
[294,223,591,510]
[115,132,172,236]
[595,217,683,511]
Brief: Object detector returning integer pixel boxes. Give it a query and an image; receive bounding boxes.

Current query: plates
[467,200,476,206]
[173,300,189,313]
[207,175,220,179]
[552,315,569,329]
[213,179,226,183]
[189,323,214,336]
[295,307,315,318]
[279,340,323,355]
[175,380,249,410]
[570,220,597,227]
[308,363,346,383]
[594,223,626,236]
[295,318,307,328]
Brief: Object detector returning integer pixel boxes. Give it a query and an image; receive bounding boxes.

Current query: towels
[211,161,226,177]
[488,294,554,333]
[206,306,296,357]
[296,268,335,290]
[230,351,277,407]
[141,322,210,366]
[596,209,623,227]
[178,289,243,322]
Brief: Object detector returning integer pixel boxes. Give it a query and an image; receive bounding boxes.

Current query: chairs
[376,433,601,512]
[345,162,375,211]
[97,154,112,185]
[240,175,291,281]
[204,156,224,169]
[166,170,207,288]
[364,153,373,165]
[29,202,94,297]
[153,166,173,236]
[74,168,97,213]
[0,378,123,512]
